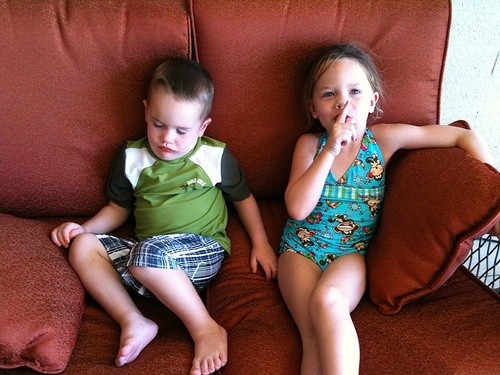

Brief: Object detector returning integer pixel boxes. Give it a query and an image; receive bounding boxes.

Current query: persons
[51,57,278,375]
[278,41,486,375]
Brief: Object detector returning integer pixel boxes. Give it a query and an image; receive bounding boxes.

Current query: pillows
[0,212,85,375]
[368,120,500,315]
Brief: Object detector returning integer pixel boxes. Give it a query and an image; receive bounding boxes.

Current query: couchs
[0,0,500,375]
